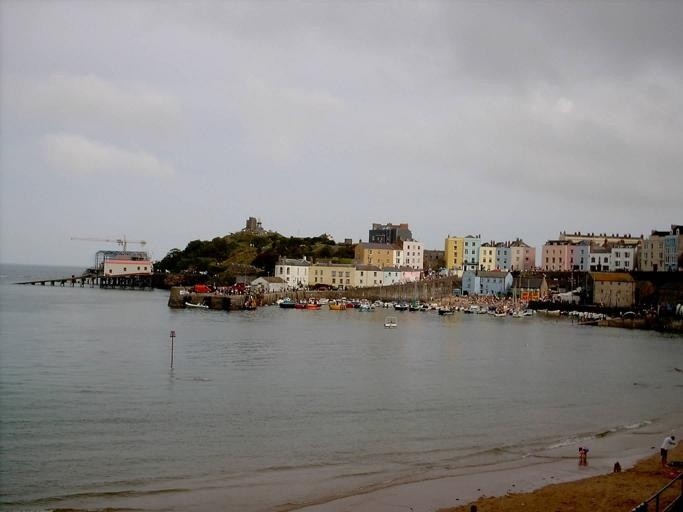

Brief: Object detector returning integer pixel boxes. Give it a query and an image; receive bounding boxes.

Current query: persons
[581,449,588,464]
[435,288,524,316]
[659,436,676,468]
[577,447,589,465]
[214,282,300,299]
[613,461,621,473]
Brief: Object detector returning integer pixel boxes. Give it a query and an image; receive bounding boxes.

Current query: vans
[194,285,211,293]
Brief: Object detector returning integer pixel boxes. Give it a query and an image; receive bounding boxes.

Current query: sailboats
[241,278,535,318]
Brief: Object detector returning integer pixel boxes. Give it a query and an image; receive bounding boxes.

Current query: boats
[184,300,209,310]
[384,315,398,329]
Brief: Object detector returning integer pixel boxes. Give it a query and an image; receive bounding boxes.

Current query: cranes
[70,231,147,252]
